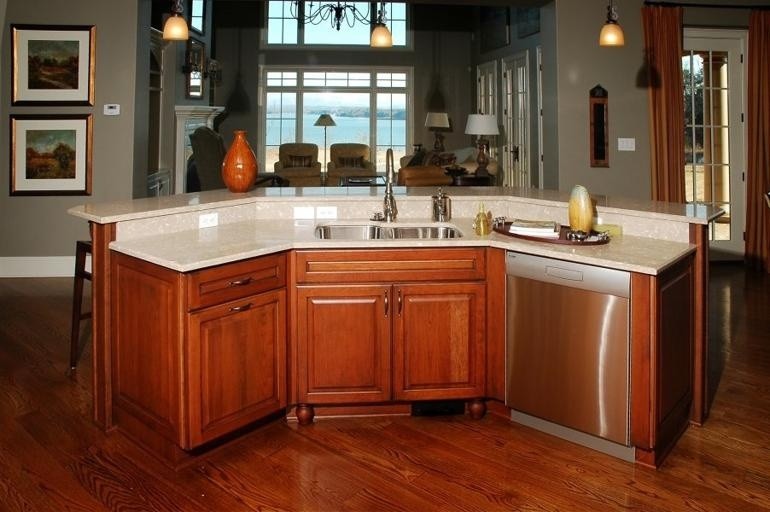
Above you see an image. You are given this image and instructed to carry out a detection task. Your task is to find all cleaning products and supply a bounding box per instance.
[431,188,450,222]
[476,200,490,237]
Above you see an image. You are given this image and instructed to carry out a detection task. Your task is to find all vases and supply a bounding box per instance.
[222,129,258,193]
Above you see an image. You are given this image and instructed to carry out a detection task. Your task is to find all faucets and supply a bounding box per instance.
[383,149,396,221]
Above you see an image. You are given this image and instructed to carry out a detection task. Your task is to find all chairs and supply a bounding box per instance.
[327,143,376,186]
[274,143,321,186]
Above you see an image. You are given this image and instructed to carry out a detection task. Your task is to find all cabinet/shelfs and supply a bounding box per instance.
[111,249,291,473]
[291,247,505,426]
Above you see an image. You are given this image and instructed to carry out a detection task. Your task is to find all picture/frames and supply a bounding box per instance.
[479,13,511,53]
[9,113,93,196]
[10,23,96,106]
[186,36,206,99]
[515,6,539,39]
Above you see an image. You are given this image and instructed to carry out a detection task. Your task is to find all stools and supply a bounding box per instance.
[70,240,92,368]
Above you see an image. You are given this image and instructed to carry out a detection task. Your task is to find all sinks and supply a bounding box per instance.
[313,223,464,240]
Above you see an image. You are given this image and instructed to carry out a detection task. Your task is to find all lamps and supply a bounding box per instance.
[599,0,625,47]
[464,114,500,176]
[424,112,449,165]
[290,0,393,48]
[313,114,336,176]
[163,0,189,41]
